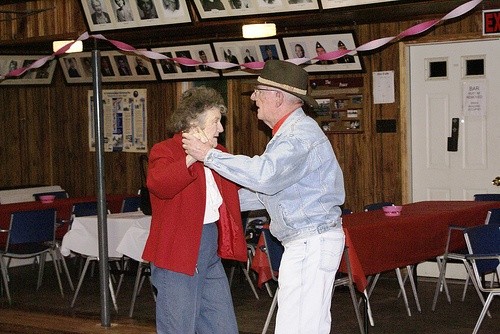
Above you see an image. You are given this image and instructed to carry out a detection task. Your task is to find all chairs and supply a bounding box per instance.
[0,191,157,318]
[240,194,500,334]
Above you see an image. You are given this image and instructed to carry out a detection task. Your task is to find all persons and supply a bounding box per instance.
[142,86,248,334]
[89,0,184,25]
[64,45,277,78]
[6,59,53,80]
[182,59,346,334]
[295,41,356,66]
[200,0,312,13]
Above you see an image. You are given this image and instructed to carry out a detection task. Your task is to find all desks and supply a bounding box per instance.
[60,210,159,312]
[251,201,500,334]
[0,185,69,204]
[0,194,123,269]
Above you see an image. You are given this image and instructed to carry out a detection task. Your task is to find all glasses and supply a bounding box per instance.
[253,88,280,94]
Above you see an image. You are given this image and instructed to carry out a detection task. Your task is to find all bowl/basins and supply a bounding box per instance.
[383,206,402,216]
[39,195,56,203]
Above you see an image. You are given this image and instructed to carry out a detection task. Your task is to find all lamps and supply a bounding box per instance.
[53,39,83,53]
[242,22,276,38]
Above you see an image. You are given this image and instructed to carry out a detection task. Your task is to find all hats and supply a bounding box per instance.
[199,51,205,55]
[265,46,272,51]
[316,41,323,48]
[240,59,319,108]
[337,41,345,47]
[246,49,249,52]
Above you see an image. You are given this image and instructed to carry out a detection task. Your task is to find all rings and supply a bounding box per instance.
[184,144,186,149]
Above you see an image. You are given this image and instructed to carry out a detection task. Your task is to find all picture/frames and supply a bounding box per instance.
[483,9,500,36]
[0,0,423,89]
